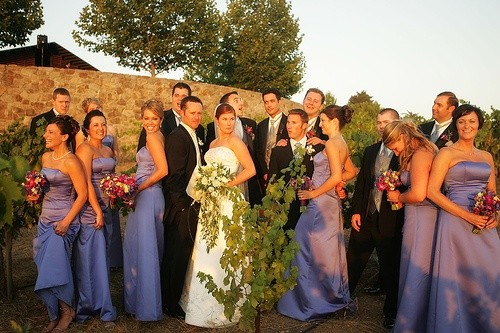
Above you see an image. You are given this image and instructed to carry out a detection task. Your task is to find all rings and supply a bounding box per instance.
[301,195,302,198]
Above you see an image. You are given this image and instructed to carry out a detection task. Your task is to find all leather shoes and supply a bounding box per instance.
[364,284,389,294]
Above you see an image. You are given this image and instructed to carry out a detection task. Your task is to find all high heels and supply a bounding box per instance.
[41,307,76,333]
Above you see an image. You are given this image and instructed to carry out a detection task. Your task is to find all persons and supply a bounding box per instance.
[201,89,360,322]
[130,81,256,333]
[29,87,125,333]
[347,90,500,333]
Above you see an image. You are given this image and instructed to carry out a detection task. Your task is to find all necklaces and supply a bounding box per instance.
[460,145,475,158]
[51,149,71,161]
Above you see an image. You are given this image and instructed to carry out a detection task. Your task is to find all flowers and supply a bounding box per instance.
[472,191,500,234]
[306,145,315,161]
[441,130,453,142]
[307,126,317,138]
[100,173,138,216]
[244,123,255,140]
[20,169,48,206]
[193,162,243,254]
[374,169,404,210]
[290,176,315,212]
[197,137,204,146]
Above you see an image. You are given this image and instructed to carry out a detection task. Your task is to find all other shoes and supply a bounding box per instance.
[383,314,396,328]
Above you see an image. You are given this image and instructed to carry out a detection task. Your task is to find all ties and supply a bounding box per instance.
[174,113,181,123]
[430,120,452,143]
[269,114,282,139]
[378,144,389,165]
[293,143,302,161]
